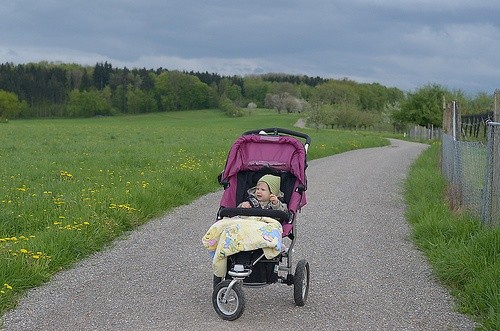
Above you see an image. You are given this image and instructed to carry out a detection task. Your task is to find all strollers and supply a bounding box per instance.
[212,127,310,321]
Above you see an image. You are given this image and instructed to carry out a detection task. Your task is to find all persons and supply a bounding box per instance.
[237,174,288,224]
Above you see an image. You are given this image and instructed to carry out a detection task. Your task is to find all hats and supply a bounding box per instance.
[258,174,281,195]
